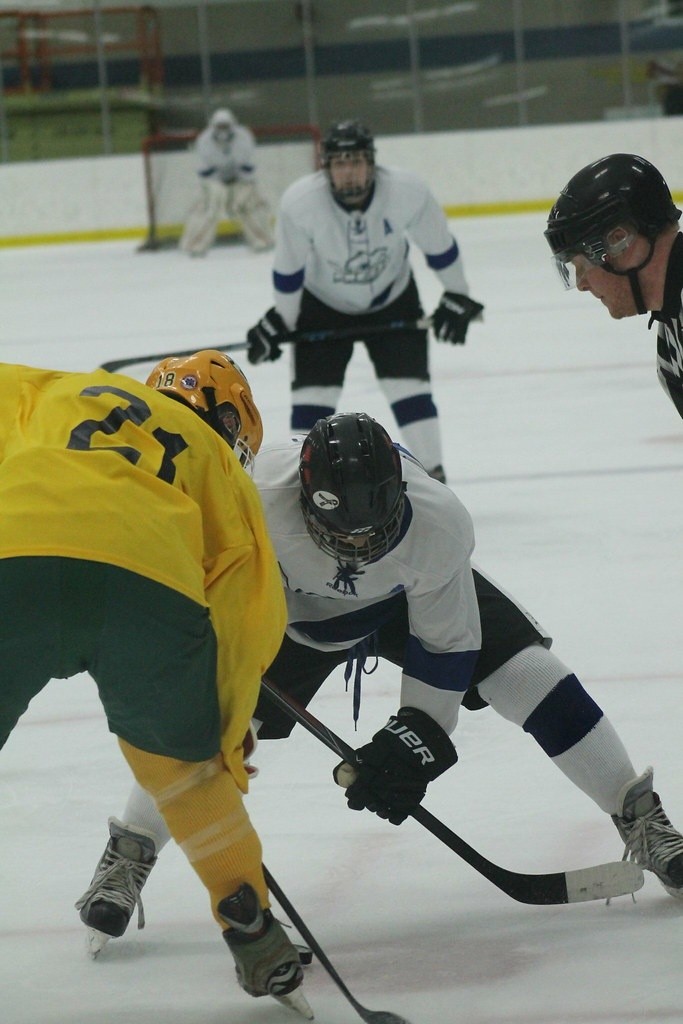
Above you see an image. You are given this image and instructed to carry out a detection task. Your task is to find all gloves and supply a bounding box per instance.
[429,291,484,345]
[333,706,458,826]
[246,307,289,365]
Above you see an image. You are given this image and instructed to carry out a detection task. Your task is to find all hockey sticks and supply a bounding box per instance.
[260,673,647,907]
[98,309,485,374]
[262,862,408,1024]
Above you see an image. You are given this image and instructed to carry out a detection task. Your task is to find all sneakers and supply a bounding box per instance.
[605,765,683,905]
[219,882,314,1021]
[74,815,160,961]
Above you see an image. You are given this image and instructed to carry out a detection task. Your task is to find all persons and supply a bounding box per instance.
[180,108,274,260]
[657,48,683,115]
[544,154,683,418]
[74,413,683,964]
[0,349,315,1022]
[247,121,471,484]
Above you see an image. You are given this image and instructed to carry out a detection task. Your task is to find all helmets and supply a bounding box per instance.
[213,109,235,143]
[145,350,264,481]
[298,412,408,571]
[319,118,376,204]
[544,153,682,292]
